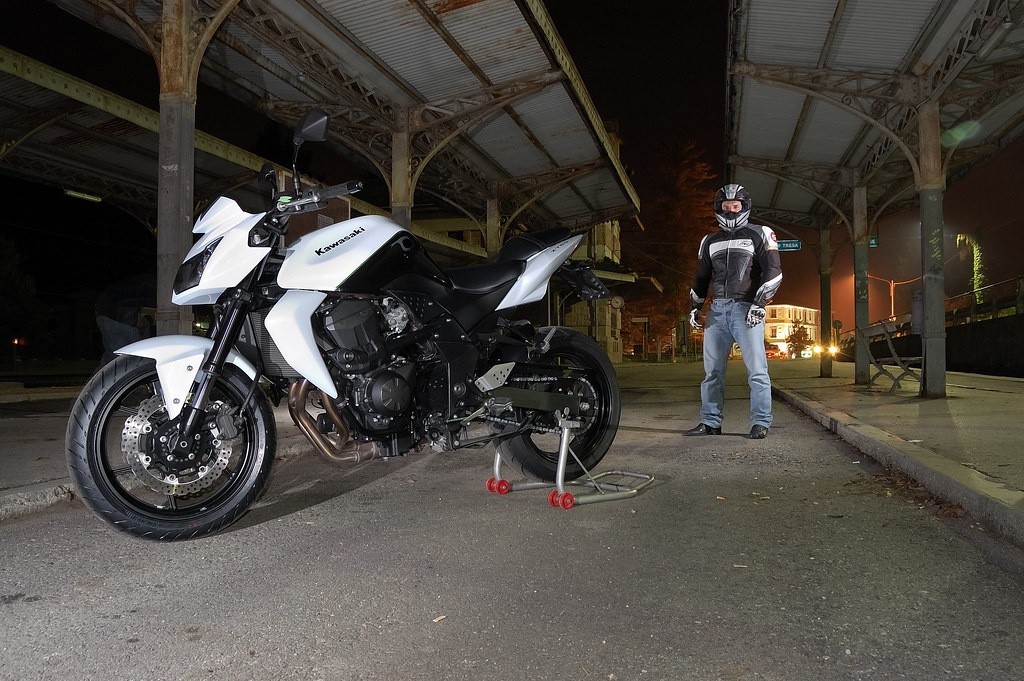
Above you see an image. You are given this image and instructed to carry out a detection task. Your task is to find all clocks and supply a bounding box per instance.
[611,295,624,309]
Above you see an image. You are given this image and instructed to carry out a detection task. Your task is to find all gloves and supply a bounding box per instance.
[745,302,766,328]
[689,309,704,331]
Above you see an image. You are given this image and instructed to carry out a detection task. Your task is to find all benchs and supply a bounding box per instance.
[855,321,923,392]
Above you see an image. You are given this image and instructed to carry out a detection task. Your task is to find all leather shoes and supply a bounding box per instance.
[682,423,721,436]
[749,425,768,438]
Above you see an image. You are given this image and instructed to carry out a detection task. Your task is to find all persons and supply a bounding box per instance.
[686,184,782,438]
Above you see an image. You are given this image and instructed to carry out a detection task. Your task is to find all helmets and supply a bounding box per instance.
[713,183,752,233]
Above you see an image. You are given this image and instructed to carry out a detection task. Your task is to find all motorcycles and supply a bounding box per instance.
[65,105,654,543]
